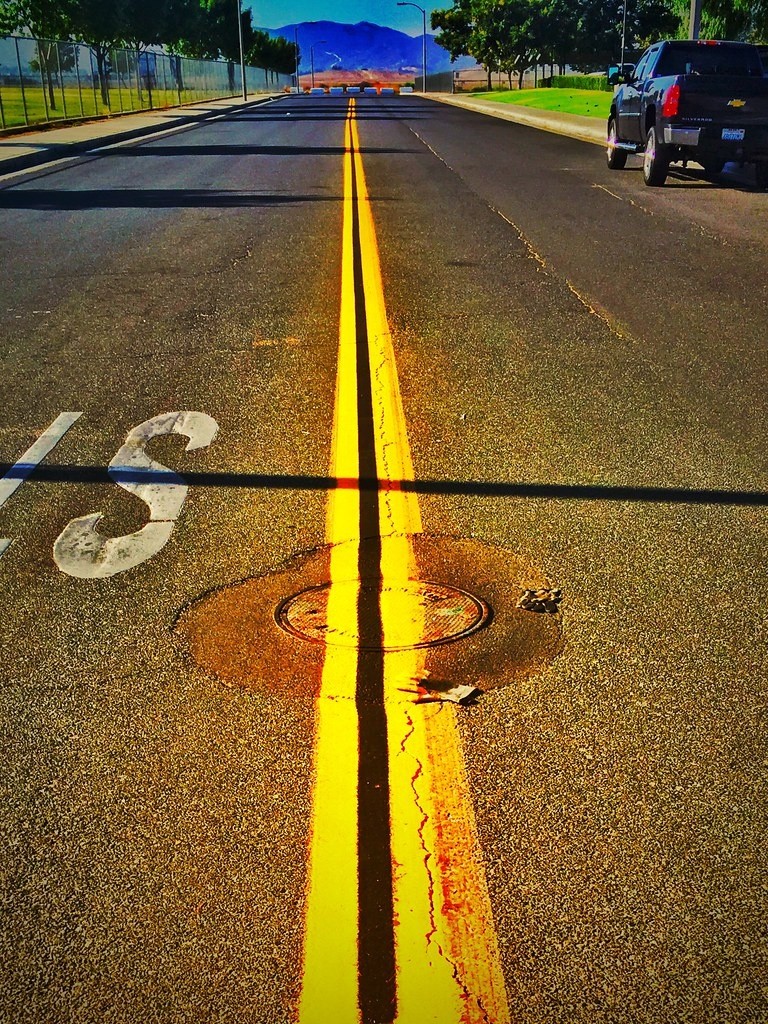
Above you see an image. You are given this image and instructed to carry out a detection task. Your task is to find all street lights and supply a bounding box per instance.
[397,2,426,94]
[295,21,318,94]
[309,40,327,88]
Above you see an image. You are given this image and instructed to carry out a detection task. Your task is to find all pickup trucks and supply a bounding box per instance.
[607,39,768,186]
